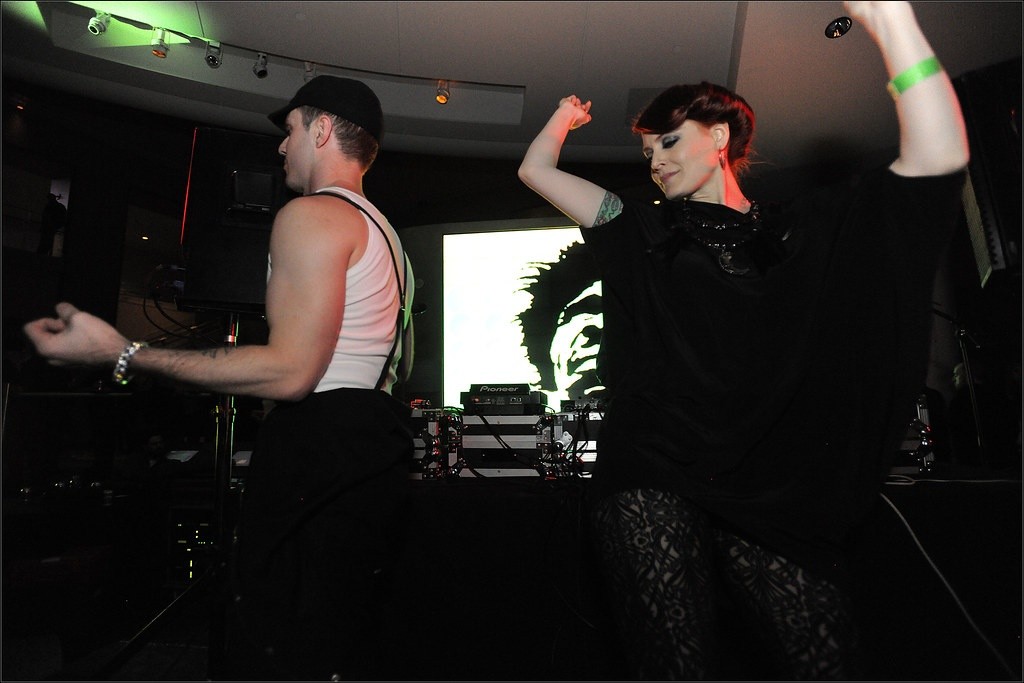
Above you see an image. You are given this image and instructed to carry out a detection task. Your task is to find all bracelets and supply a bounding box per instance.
[888,55,942,102]
[113,341,149,385]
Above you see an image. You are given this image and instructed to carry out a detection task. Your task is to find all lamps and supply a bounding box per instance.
[252,60,269,80]
[151,29,170,58]
[88,12,111,36]
[435,80,450,104]
[205,41,224,69]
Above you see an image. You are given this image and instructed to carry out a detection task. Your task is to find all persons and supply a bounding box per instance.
[25,76,416,682]
[517,3,969,682]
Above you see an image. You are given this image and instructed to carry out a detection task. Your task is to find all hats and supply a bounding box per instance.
[267,75,385,145]
